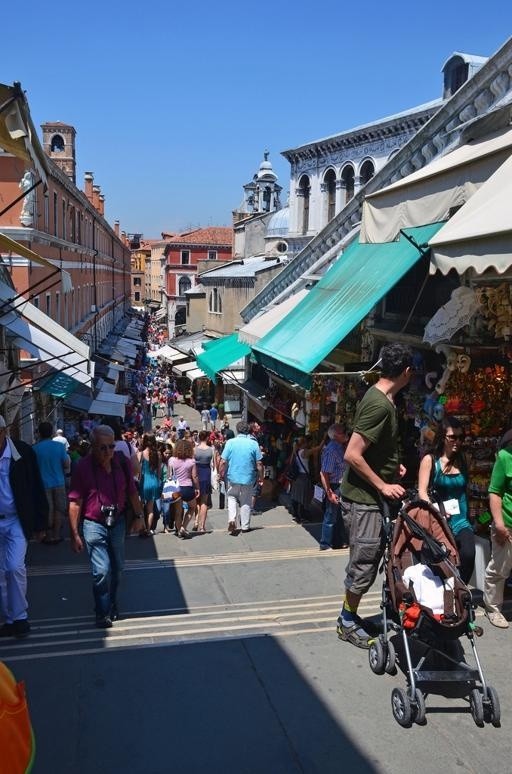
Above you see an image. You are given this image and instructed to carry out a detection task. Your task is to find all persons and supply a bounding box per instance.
[127,320,218,439]
[335,340,416,650]
[31,421,91,544]
[0,415,50,640]
[417,415,477,587]
[68,425,144,627]
[482,444,512,627]
[99,417,273,538]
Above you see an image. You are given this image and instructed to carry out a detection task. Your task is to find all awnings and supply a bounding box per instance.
[42,313,146,423]
[0,233,96,392]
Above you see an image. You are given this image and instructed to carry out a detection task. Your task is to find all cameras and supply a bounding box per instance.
[101,504,120,529]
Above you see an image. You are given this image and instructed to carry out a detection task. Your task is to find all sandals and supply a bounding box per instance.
[337,619,378,648]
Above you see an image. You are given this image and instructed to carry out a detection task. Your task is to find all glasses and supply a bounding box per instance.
[449,435,465,441]
[101,445,115,450]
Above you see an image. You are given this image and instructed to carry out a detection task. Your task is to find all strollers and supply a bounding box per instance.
[366,493,506,735]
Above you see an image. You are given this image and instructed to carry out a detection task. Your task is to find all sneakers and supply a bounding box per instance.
[97,612,118,627]
[0,619,30,637]
[485,610,509,628]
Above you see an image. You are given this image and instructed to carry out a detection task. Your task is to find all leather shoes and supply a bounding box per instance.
[228,522,251,533]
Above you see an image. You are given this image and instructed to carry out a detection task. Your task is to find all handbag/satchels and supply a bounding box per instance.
[161,457,181,503]
[126,503,143,537]
[285,465,298,480]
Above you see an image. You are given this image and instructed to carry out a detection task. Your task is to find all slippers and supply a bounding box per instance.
[144,527,205,537]
